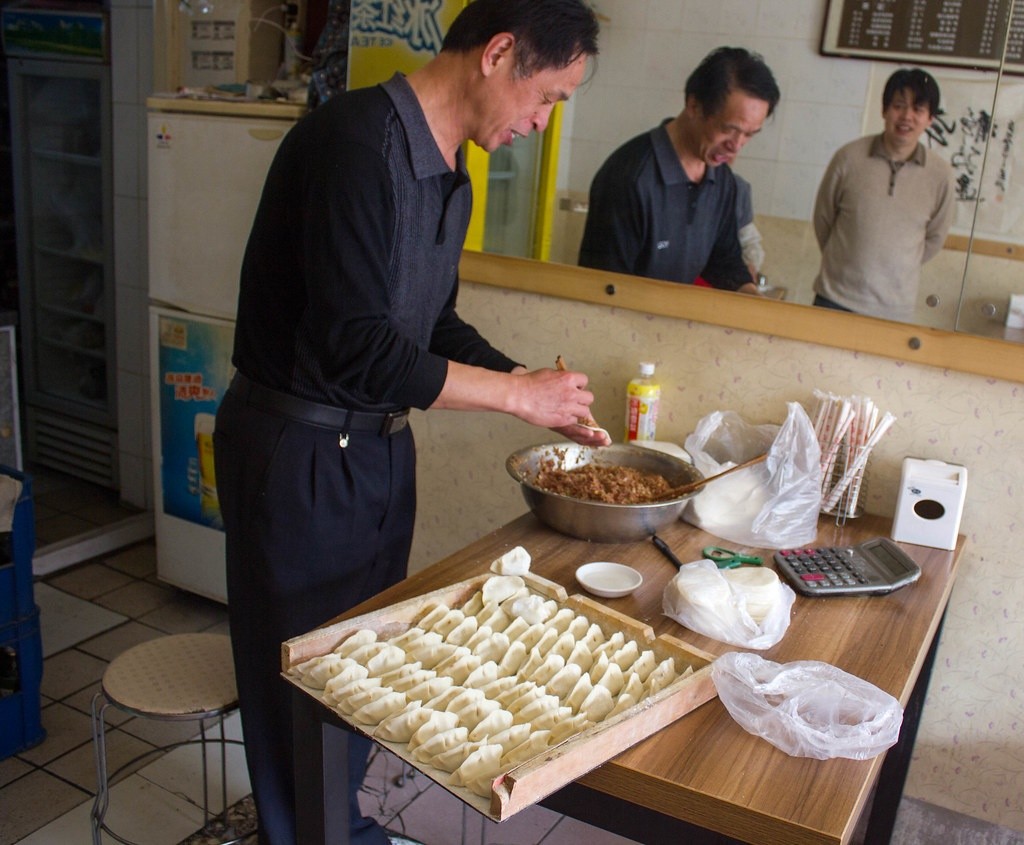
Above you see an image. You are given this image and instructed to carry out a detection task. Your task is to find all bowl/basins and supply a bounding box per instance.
[506,441,705,542]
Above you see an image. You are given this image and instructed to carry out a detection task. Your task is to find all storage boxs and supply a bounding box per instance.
[0,606,49,762]
[0,464,39,631]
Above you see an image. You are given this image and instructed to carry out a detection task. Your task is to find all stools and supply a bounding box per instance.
[89,631,257,845]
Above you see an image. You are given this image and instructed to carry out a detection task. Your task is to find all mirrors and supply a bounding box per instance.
[457,0,1024,384]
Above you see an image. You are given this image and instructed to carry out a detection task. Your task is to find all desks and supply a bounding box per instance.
[285,508,966,845]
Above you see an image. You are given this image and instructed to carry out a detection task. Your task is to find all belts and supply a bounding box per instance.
[227,374,412,438]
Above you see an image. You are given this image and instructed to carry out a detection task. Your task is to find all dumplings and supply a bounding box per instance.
[286,545,695,799]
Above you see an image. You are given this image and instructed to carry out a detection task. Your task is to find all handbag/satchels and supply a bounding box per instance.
[660,558,797,651]
[678,400,822,550]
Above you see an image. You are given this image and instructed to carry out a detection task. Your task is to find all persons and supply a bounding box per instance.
[580,44,779,292]
[813,69,956,325]
[219,0,619,845]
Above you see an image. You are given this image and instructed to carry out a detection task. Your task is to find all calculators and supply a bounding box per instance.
[772,535,923,598]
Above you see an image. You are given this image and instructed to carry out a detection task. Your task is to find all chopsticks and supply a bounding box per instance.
[555,355,567,371]
[811,392,896,515]
[655,452,767,501]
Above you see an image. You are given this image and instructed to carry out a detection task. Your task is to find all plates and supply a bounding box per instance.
[575,562,643,598]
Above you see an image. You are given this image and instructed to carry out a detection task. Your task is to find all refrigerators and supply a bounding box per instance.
[0,7,118,491]
[342,0,567,263]
[145,97,304,604]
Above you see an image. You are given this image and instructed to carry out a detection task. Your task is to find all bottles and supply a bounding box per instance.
[623,361,659,444]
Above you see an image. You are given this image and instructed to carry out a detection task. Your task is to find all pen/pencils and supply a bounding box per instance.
[653,534,683,571]
